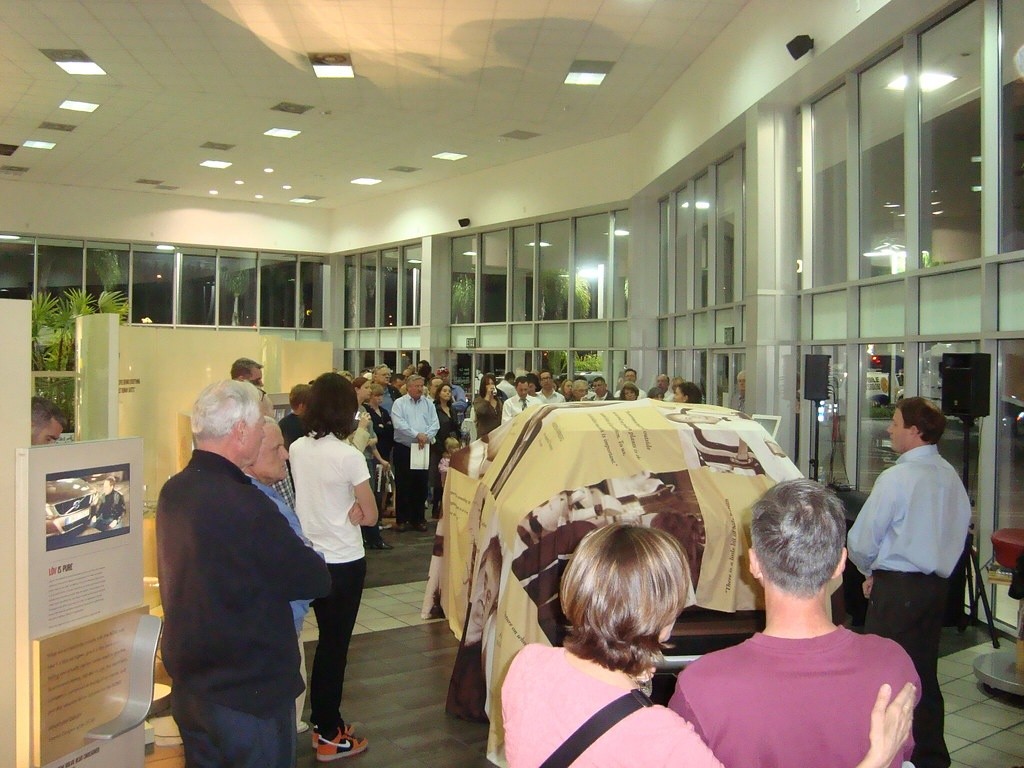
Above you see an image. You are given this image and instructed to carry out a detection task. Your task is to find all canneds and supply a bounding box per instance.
[492,385,497,395]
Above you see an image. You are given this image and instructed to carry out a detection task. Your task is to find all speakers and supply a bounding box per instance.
[803,353,832,403]
[938,352,991,418]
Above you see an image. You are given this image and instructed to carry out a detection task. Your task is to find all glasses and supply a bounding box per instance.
[258,388,267,402]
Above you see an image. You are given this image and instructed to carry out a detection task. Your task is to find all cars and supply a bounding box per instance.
[46,478,100,539]
[440,402,856,717]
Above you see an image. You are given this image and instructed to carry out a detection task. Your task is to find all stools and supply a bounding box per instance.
[973,528,1024,697]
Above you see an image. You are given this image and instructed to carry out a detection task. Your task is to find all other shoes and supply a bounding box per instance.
[432,512,440,518]
[395,523,406,532]
[415,524,427,531]
[370,540,392,549]
[297,721,309,733]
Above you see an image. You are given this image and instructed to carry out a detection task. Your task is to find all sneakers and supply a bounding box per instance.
[317,727,369,761]
[312,724,354,748]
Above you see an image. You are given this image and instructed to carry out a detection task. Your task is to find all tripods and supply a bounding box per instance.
[944,417,1001,650]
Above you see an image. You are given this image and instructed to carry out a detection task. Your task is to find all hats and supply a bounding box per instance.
[437,366,449,375]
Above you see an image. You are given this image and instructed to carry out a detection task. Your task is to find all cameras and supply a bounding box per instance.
[355,412,363,420]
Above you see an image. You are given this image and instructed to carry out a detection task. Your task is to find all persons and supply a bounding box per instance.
[244,414,323,733]
[155,379,332,768]
[289,373,377,761]
[849,396,972,768]
[279,361,701,551]
[501,521,916,768]
[96,477,125,532]
[669,480,922,768]
[731,370,746,412]
[231,357,265,390]
[255,395,295,505]
[32,397,65,447]
